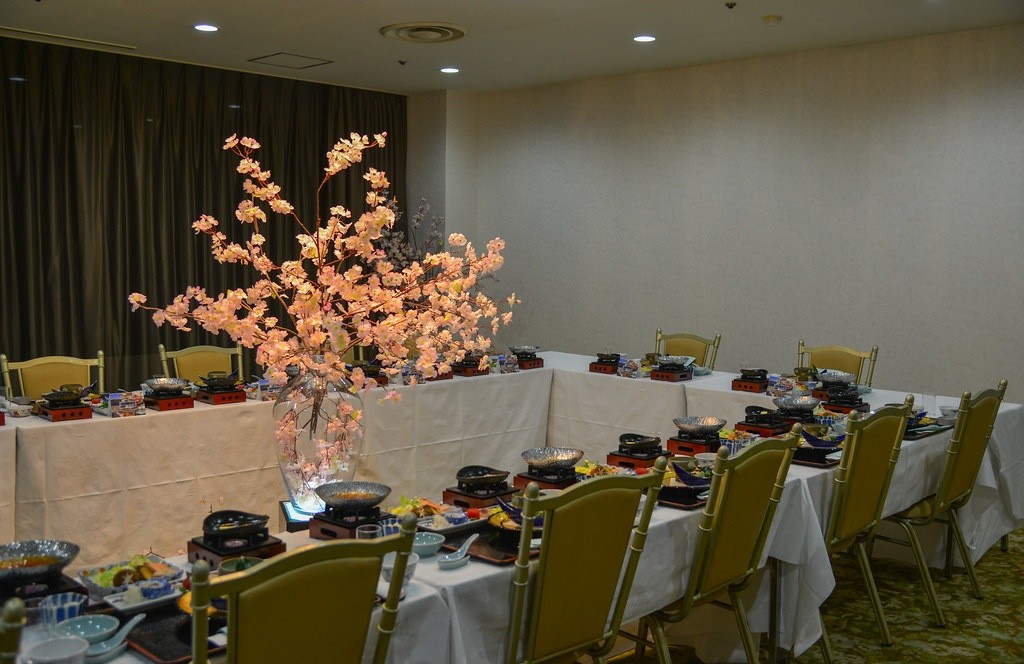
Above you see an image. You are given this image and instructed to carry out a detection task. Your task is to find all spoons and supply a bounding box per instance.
[439,533,477,561]
[85,614,147,656]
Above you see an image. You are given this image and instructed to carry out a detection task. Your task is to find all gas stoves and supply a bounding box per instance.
[0,355,870,617]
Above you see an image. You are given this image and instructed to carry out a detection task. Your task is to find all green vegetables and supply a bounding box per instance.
[696,466,713,476]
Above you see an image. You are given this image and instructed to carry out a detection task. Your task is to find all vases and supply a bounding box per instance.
[273,354,368,516]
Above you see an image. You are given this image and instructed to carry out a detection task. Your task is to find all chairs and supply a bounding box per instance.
[634,421,803,664]
[159,340,244,385]
[503,454,668,664]
[818,393,915,664]
[652,328,721,371]
[0,349,105,402]
[866,377,1008,627]
[189,514,419,664]
[795,340,878,389]
[314,334,365,366]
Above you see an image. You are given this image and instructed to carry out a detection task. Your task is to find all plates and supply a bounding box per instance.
[662,477,710,490]
[488,510,545,532]
[104,585,183,615]
[177,590,229,623]
[418,512,486,534]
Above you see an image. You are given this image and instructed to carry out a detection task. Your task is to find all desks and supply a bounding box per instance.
[0,349,1024,664]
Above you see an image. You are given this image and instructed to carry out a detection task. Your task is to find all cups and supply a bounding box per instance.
[354,525,381,540]
[39,592,88,632]
[377,519,402,536]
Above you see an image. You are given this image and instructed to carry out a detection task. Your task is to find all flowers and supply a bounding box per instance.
[126,131,522,507]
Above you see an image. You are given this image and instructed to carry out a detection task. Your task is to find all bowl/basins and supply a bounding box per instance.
[217,556,263,578]
[940,405,958,417]
[55,615,118,641]
[381,551,419,585]
[696,451,718,469]
[29,636,88,664]
[908,411,928,425]
[665,456,696,474]
[512,491,546,507]
[410,532,445,557]
